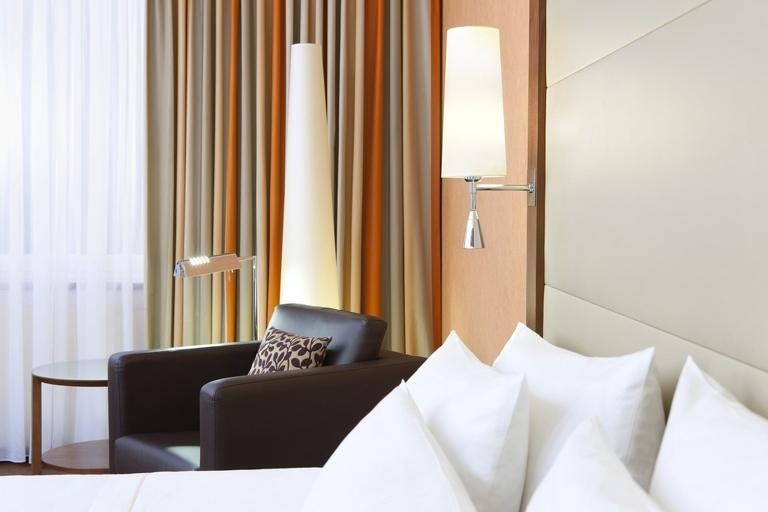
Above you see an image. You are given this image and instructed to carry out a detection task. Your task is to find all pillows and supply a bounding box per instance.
[492,319,665,512]
[320,379,476,512]
[404,330,526,512]
[648,357,768,512]
[247,327,331,375]
[526,415,660,512]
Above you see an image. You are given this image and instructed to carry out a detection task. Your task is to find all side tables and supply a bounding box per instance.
[31,359,109,474]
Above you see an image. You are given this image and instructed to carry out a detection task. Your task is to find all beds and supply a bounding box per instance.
[0,467,363,511]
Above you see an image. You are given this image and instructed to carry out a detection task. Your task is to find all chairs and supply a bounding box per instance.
[109,303,427,470]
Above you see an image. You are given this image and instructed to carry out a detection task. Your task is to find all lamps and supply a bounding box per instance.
[440,25,537,248]
[172,252,258,342]
[278,44,343,314]
